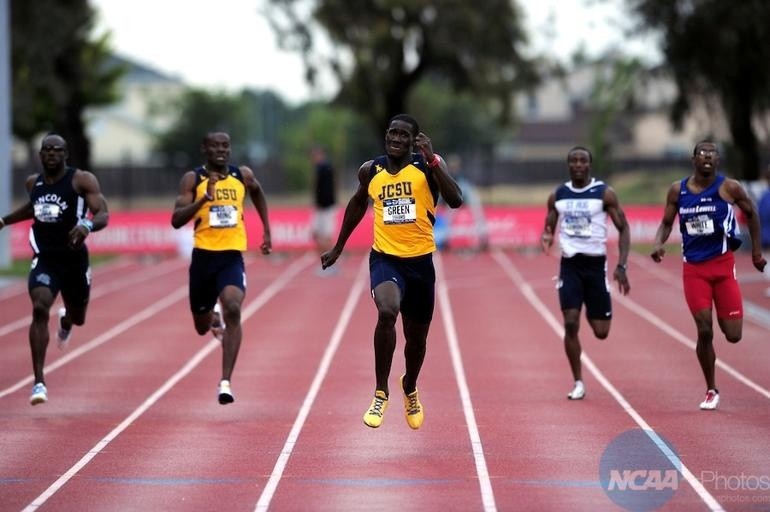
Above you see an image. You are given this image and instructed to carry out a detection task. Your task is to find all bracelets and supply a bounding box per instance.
[617,262,628,272]
[204,192,214,201]
[78,218,96,232]
[0,217,6,227]
[753,256,764,263]
[426,153,441,169]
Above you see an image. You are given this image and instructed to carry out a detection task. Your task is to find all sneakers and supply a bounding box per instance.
[29,382,49,406]
[700,387,720,412]
[216,377,236,405]
[363,390,389,429]
[567,382,586,400]
[400,372,424,429]
[53,308,72,352]
[211,302,227,341]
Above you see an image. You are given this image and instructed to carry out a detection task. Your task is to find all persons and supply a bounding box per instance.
[649,139,769,411]
[307,144,339,278]
[538,145,633,401]
[170,129,275,407]
[320,112,465,431]
[0,130,112,407]
